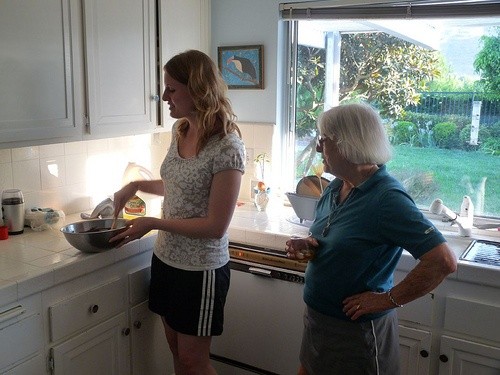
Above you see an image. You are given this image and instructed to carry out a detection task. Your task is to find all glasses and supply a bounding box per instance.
[315,135,328,148]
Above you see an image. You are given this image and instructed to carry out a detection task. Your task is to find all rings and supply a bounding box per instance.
[352,304,360,312]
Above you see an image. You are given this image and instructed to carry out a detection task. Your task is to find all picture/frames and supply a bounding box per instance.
[217,44,264,90]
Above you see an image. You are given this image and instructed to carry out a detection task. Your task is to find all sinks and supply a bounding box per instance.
[459,239,500,267]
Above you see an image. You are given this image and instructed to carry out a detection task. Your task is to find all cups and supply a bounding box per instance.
[0,220,8,240]
[291,232,313,263]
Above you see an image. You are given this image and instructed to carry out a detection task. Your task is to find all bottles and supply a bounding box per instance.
[255,182,269,211]
[123,162,156,219]
[2,189,24,235]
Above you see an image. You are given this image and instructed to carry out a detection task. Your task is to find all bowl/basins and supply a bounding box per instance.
[60,218,127,253]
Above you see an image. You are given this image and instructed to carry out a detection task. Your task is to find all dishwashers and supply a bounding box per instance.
[210,259,306,375]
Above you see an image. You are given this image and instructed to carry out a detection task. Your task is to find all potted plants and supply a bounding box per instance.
[253,152,272,214]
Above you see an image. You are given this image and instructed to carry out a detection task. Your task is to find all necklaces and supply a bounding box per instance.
[322,166,376,237]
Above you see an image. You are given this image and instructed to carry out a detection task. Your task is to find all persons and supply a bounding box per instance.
[285,104,457,375]
[108,51,245,375]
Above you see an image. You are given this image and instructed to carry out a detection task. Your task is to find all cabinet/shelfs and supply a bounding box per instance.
[0,250,175,375]
[394,270,500,375]
[0,0,211,149]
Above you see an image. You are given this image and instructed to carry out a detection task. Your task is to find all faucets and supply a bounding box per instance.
[456,174,487,216]
[429,195,474,237]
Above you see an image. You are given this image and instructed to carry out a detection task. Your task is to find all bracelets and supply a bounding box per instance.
[387,288,403,308]
[132,181,138,191]
